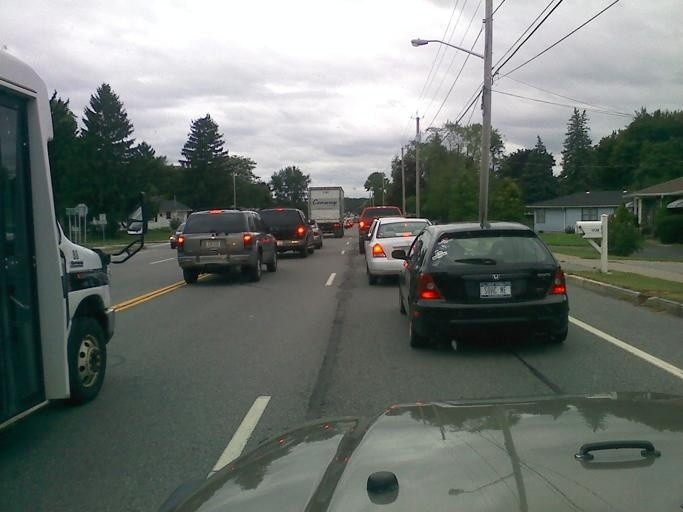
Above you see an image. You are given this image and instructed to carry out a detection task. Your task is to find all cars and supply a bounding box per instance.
[393,218,569,350]
[310,223,323,249]
[362,217,435,285]
[158,390,683,512]
[169,222,186,249]
[343,216,360,229]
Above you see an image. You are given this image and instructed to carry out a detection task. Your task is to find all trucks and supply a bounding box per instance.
[0,49,148,433]
[307,186,345,238]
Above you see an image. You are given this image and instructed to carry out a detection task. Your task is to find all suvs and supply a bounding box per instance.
[260,208,316,257]
[357,206,405,255]
[176,210,278,284]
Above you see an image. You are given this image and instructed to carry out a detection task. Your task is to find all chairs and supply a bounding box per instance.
[443,241,514,263]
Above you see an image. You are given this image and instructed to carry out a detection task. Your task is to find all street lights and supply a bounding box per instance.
[411,38,484,60]
